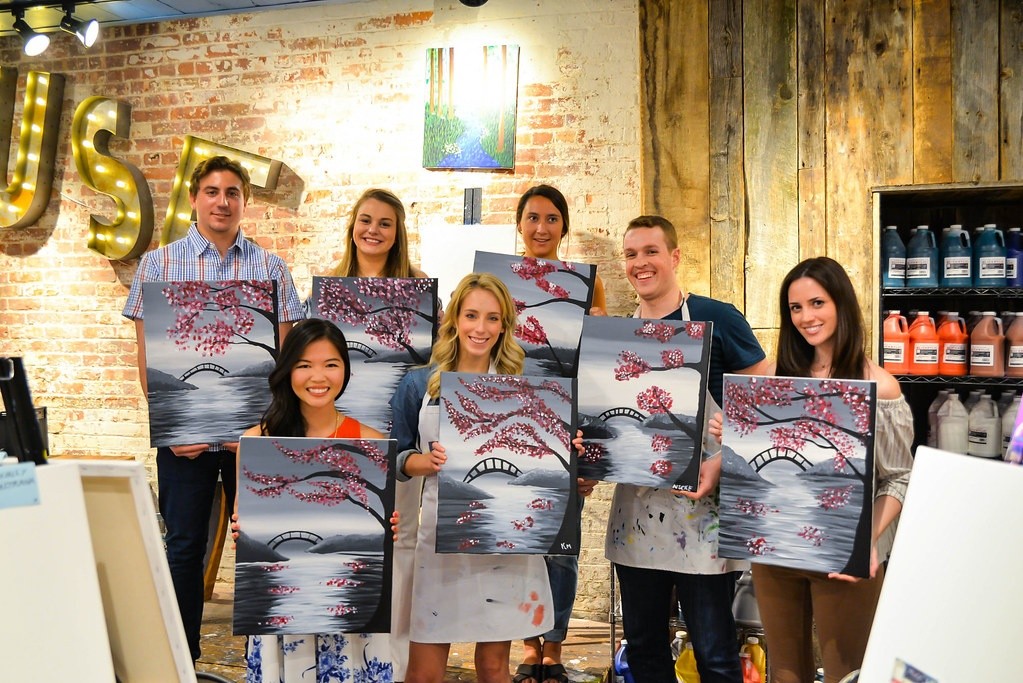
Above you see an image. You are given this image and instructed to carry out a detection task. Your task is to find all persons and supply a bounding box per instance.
[121,156,303,669]
[512,185,604,683]
[230,318,393,683]
[604,216,767,683]
[388,274,587,683]
[709,257,915,683]
[303,188,443,319]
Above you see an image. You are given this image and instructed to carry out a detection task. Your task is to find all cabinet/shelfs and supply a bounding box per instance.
[870,183,1023,465]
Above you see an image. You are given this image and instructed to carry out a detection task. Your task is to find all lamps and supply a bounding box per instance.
[12,8,51,56]
[59,0,99,49]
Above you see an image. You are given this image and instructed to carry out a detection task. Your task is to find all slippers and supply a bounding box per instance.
[514,662,543,683]
[541,663,569,683]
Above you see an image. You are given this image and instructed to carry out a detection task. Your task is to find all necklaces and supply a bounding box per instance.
[822,365,825,368]
[333,408,339,437]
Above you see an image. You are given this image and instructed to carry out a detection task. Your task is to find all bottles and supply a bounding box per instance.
[814,668,824,683]
[615,639,635,683]
[881,223,1023,287]
[738,652,760,683]
[740,637,766,683]
[670,630,688,661]
[674,642,700,683]
[883,309,1023,377]
[928,388,1021,457]
